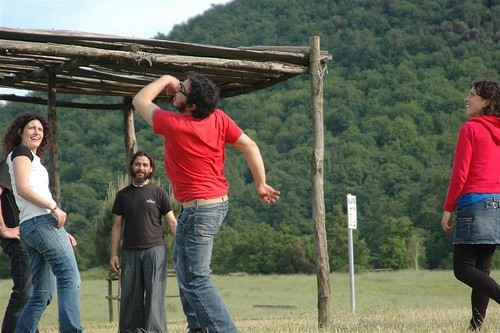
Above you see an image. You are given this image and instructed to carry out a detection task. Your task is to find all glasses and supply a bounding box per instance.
[179,84,187,97]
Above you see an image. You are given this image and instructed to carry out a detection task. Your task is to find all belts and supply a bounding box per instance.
[181,195,228,208]
